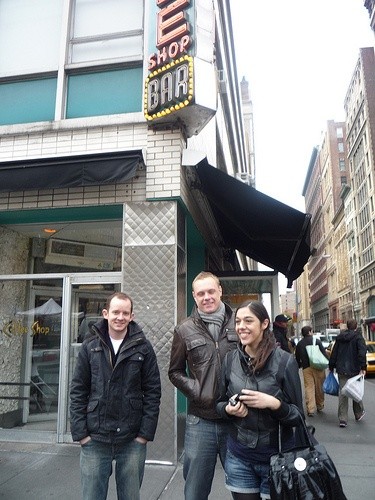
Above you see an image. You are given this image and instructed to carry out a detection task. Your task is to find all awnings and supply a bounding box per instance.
[209,164,310,289]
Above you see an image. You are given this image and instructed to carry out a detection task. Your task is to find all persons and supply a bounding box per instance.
[69,291,162,500]
[83,321,96,341]
[271,314,295,354]
[329,319,367,426]
[296,326,331,416]
[168,272,241,500]
[216,300,305,500]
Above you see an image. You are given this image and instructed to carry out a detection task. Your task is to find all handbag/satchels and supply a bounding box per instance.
[268,403,347,500]
[305,334,330,370]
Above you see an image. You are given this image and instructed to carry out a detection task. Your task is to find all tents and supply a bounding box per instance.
[17,299,83,350]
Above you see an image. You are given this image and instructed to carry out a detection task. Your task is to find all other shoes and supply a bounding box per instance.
[317,407,323,412]
[339,421,347,427]
[308,413,314,416]
[355,410,366,422]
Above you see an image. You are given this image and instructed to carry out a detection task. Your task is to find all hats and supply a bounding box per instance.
[275,314,292,322]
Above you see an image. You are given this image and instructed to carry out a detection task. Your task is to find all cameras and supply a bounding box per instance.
[229,394,240,406]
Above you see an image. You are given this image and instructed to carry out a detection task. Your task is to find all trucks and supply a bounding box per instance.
[325,328,341,341]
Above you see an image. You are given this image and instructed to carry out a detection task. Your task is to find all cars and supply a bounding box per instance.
[365,340,375,380]
[326,340,335,360]
[290,331,329,348]
